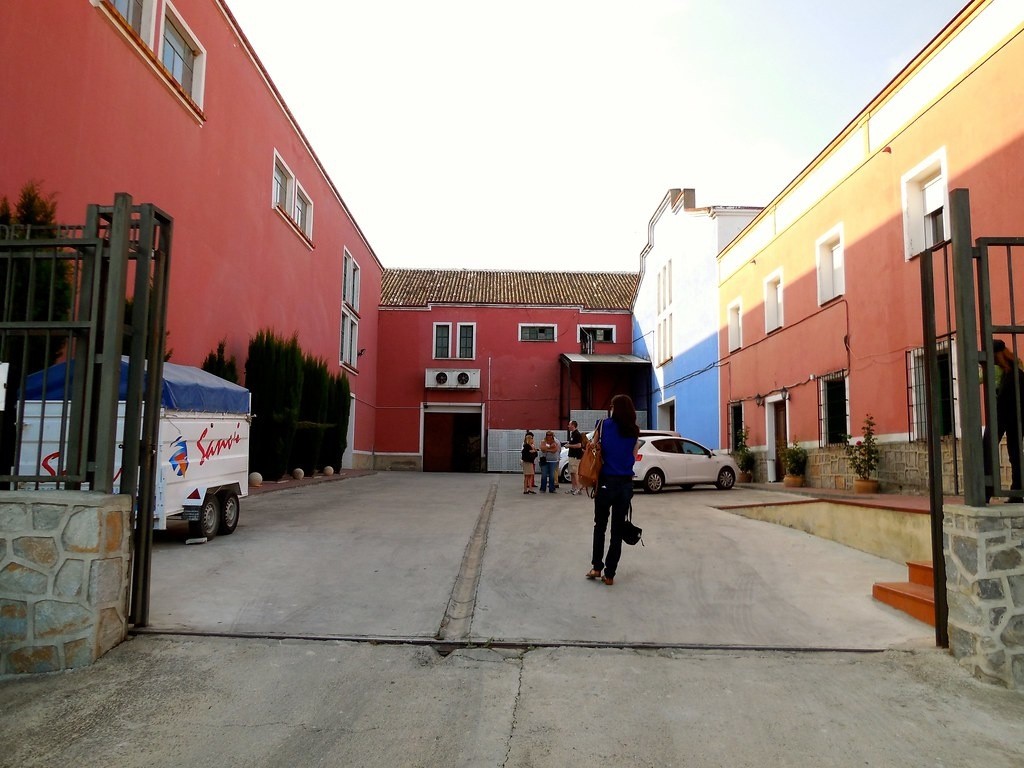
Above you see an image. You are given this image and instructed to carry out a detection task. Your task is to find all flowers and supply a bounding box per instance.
[840,410,880,480]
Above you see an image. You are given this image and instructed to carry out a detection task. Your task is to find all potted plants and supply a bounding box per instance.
[729,424,755,484]
[775,432,808,487]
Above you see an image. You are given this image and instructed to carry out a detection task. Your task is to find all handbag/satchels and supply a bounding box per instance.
[539,456,546,467]
[621,501,645,546]
[578,419,604,499]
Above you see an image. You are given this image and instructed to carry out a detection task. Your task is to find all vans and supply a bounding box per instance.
[557,429,681,483]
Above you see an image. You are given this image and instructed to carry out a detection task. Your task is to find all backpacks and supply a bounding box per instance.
[579,431,589,450]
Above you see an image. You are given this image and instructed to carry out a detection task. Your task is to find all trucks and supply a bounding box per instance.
[11,351,253,540]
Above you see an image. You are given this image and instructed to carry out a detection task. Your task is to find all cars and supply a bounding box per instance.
[632,435,742,492]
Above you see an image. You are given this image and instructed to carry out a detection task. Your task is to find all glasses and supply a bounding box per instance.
[531,438,533,439]
[546,434,552,436]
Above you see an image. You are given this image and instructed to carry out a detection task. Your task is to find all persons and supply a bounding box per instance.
[522,435,538,494]
[521,430,538,487]
[564,421,583,494]
[983,339,1024,503]
[551,431,561,488]
[539,431,558,493]
[586,395,640,585]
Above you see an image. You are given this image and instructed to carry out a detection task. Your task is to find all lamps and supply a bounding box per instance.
[754,393,765,407]
[357,348,365,355]
[779,385,790,401]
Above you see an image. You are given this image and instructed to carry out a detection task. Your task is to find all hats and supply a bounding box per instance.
[526,431,534,436]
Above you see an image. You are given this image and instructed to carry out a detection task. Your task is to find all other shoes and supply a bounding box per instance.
[553,489,558,493]
[524,490,537,494]
[547,484,559,488]
[532,484,538,487]
[564,488,582,495]
[585,568,601,577]
[600,574,613,585]
[538,489,546,493]
[526,485,529,488]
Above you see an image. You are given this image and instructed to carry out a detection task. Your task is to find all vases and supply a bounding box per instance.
[854,477,880,494]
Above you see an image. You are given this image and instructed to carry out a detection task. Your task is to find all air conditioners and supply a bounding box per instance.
[450,369,480,389]
[424,368,450,388]
